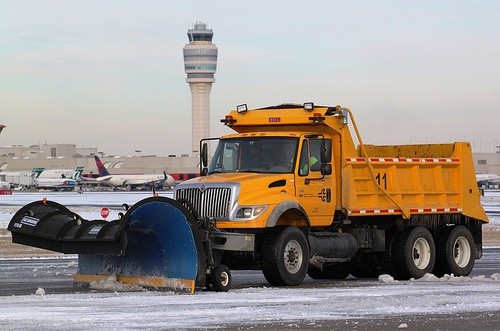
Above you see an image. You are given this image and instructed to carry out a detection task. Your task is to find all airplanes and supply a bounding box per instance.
[31,154,176,191]
[475,173,500,188]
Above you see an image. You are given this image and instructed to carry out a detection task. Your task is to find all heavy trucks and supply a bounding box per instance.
[174,101,489,286]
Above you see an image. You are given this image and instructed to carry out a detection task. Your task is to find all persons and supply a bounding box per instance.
[286,139,321,174]
[241,143,271,170]
